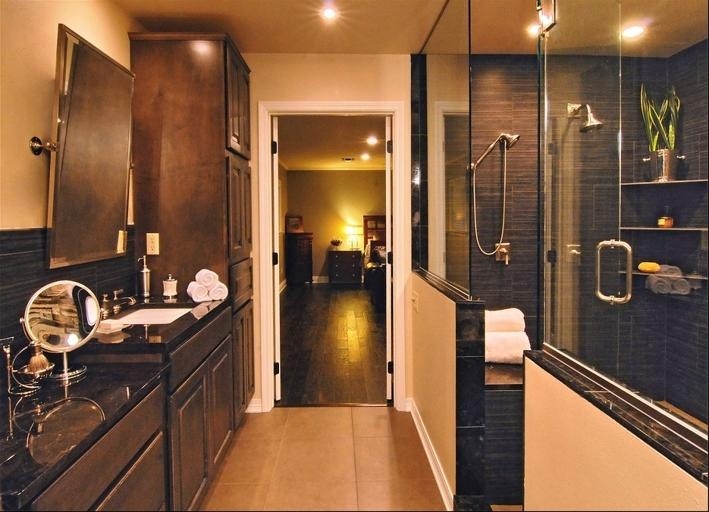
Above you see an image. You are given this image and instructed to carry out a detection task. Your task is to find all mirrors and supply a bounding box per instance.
[25,386,105,472]
[46,22,135,270]
[19,278,102,386]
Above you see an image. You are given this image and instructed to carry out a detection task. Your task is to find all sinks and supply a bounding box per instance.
[109,302,201,324]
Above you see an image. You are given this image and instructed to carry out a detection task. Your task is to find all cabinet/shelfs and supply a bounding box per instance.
[223,41,252,161]
[166,305,238,511]
[232,257,255,413]
[284,233,313,284]
[0,361,167,509]
[226,154,255,263]
[620,177,707,408]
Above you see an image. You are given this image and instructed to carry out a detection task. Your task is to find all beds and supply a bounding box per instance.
[362,215,386,311]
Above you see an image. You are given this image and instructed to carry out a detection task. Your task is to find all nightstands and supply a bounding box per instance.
[327,250,362,288]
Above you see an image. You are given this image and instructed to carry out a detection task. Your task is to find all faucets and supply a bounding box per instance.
[98,293,137,315]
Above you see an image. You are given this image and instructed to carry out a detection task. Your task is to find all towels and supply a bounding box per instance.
[186,268,228,302]
[644,263,692,296]
[484,308,532,364]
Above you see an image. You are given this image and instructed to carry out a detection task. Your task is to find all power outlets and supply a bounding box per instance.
[146,232,160,256]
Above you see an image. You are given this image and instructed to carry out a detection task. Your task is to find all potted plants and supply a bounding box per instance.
[640,81,681,182]
